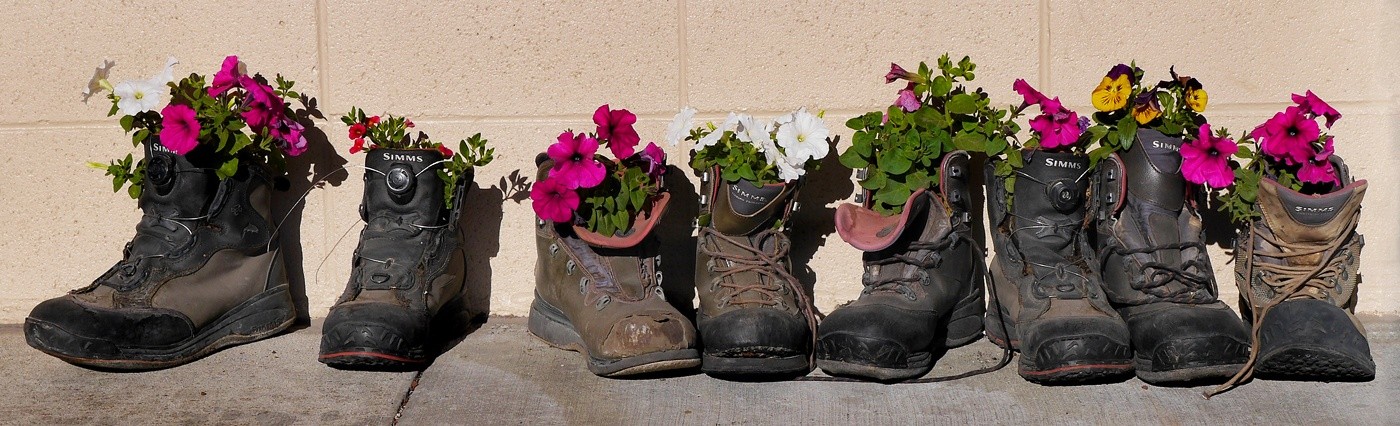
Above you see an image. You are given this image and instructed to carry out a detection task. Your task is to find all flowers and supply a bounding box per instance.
[68,42,1367,239]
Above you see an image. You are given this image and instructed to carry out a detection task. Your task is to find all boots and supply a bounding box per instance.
[692,162,816,377]
[528,151,700,377]
[984,149,1134,381]
[317,146,474,366]
[792,150,1011,384]
[24,149,295,369]
[1199,154,1377,401]
[1095,124,1251,383]
